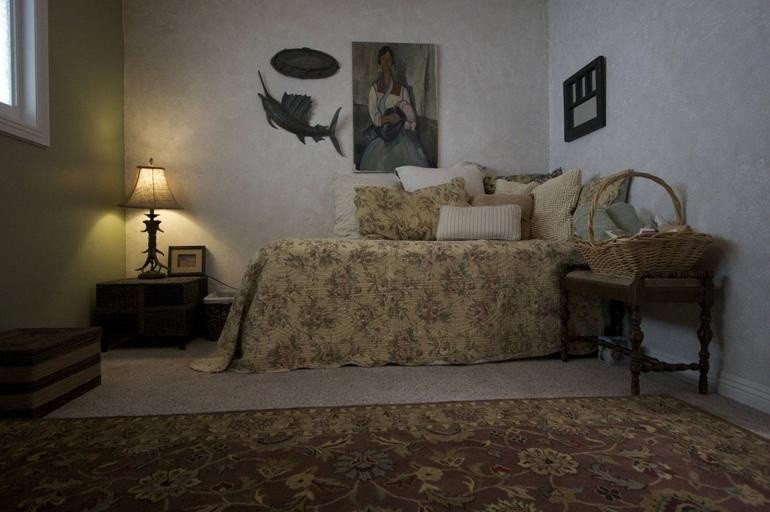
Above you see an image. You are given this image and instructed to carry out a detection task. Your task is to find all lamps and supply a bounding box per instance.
[113,156,185,279]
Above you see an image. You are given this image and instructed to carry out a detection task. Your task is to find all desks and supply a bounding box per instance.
[557,266,717,398]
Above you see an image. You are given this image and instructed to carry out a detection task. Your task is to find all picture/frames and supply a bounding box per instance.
[167,245,206,276]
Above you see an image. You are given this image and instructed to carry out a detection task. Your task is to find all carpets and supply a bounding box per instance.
[0,390,770,511]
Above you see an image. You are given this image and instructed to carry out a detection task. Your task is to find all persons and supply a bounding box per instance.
[359,46,429,170]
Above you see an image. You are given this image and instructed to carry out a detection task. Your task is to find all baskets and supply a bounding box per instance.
[576,171,713,280]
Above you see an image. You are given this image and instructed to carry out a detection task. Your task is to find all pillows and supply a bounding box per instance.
[529,168,582,242]
[483,167,562,195]
[471,192,536,242]
[354,177,470,241]
[494,178,541,195]
[394,161,486,203]
[576,167,634,212]
[436,204,522,240]
[334,172,404,240]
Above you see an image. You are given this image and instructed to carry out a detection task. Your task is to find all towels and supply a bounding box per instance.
[604,200,645,237]
[571,200,622,244]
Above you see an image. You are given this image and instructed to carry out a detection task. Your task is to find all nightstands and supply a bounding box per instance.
[97,275,209,352]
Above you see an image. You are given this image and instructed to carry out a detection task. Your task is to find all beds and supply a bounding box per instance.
[188,168,636,373]
